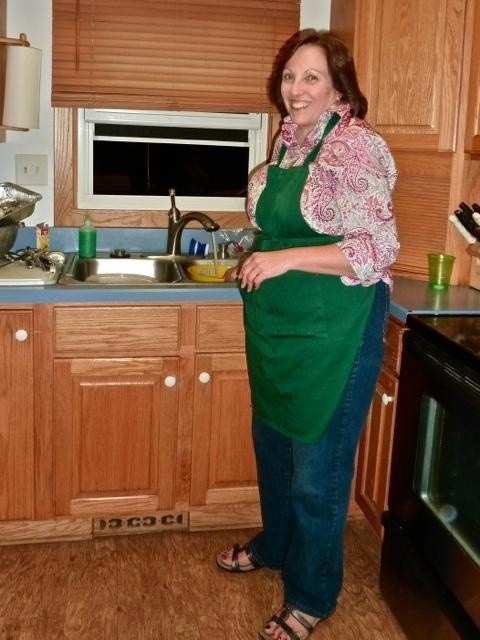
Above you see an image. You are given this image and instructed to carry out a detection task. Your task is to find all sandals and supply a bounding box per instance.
[260,602,336,640]
[216,542,264,573]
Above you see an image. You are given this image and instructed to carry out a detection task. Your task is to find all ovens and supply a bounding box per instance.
[381,330,480,634]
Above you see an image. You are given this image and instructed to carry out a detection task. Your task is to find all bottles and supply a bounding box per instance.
[78,211,97,258]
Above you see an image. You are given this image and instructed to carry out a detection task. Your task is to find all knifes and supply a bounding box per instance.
[448,199,480,246]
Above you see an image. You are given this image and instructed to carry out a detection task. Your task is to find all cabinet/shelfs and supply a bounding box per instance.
[330,0,467,154]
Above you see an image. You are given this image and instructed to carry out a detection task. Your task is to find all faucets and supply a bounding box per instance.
[217,240,241,259]
[164,188,221,254]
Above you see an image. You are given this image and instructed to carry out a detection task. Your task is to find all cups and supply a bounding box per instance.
[427,253,455,292]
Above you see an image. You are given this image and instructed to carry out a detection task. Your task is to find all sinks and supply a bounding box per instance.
[178,250,242,287]
[58,251,184,288]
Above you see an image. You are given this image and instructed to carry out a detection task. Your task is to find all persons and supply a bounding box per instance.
[216,28,400,640]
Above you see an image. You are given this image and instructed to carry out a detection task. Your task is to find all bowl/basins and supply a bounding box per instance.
[187,264,234,282]
